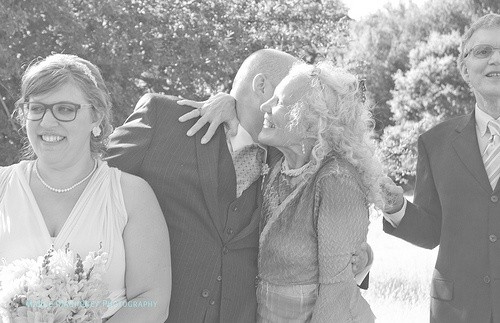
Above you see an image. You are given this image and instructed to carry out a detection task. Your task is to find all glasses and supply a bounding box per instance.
[19,97,92,122]
[463,44,500,59]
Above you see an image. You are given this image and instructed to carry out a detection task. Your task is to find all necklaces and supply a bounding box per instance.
[34,156,97,192]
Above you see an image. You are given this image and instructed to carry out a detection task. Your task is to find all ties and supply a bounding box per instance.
[230,144,264,199]
[481,120,500,192]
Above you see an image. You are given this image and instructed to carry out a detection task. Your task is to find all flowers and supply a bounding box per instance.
[0,239,111,323]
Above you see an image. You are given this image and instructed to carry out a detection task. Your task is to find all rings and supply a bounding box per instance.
[197,106,203,116]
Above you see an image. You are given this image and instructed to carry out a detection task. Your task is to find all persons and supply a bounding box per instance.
[368,14,500,323]
[176,61,378,323]
[0,54,172,323]
[101,48,375,323]
